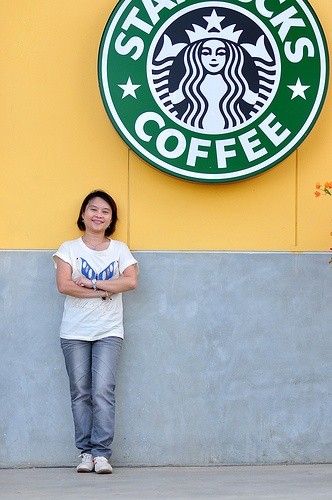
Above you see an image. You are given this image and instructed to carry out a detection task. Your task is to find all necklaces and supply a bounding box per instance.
[82,236,110,250]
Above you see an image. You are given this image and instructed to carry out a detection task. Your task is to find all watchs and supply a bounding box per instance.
[91,278,97,290]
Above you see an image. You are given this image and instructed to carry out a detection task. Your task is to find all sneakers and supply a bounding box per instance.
[77,454,94,472]
[94,456,113,473]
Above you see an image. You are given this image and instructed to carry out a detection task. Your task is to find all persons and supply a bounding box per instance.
[50,188,140,475]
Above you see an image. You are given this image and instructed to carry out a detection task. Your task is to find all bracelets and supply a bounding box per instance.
[99,289,108,300]
